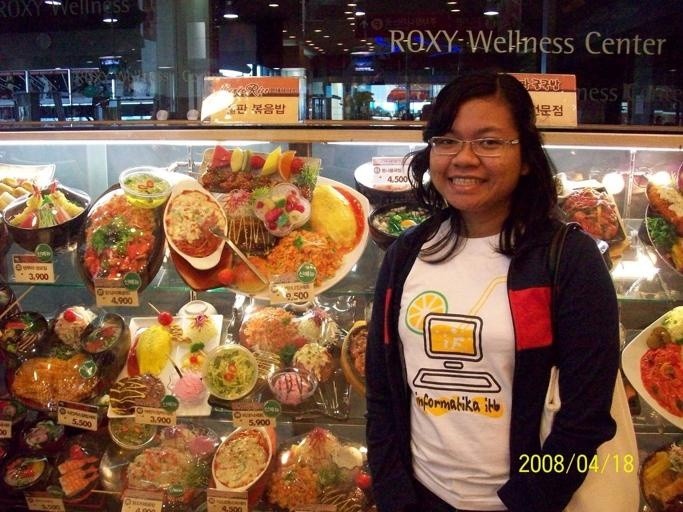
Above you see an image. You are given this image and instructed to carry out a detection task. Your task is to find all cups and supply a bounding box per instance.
[12,89,41,122]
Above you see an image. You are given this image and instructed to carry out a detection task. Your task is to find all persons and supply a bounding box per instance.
[363,69,621,512]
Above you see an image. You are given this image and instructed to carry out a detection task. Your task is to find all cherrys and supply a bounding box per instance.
[291,158,303,173]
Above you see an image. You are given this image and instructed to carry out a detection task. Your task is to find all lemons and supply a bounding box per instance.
[230,147,243,172]
[406,290,448,334]
[261,145,281,176]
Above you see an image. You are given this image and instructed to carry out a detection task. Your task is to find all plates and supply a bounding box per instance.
[620,303,683,432]
[107,313,224,418]
[76,182,166,296]
[163,179,229,272]
[169,176,372,302]
[2,310,132,413]
[211,426,273,492]
[97,420,221,509]
[266,435,374,511]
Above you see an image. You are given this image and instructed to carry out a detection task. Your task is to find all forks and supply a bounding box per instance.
[207,223,269,285]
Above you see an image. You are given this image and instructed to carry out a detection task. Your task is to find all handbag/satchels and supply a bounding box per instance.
[541,360,639,511]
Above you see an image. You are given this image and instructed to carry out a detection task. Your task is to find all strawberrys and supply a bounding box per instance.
[251,155,266,169]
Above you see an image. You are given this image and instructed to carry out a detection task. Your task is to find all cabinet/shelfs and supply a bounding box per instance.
[0,68,683,512]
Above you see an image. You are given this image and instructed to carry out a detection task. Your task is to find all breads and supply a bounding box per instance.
[108,374,164,414]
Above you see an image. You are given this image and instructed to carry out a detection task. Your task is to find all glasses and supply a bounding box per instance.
[430,136,522,157]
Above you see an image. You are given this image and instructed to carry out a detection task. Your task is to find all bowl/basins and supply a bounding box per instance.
[268,366,319,406]
[2,188,93,254]
[19,416,69,455]
[201,343,259,401]
[354,161,431,207]
[119,166,172,209]
[0,449,48,494]
[108,419,159,451]
[367,201,442,252]
[175,299,218,341]
[79,314,125,357]
[0,309,49,360]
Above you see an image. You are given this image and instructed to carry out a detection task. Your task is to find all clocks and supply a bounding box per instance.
[35,32,52,50]
